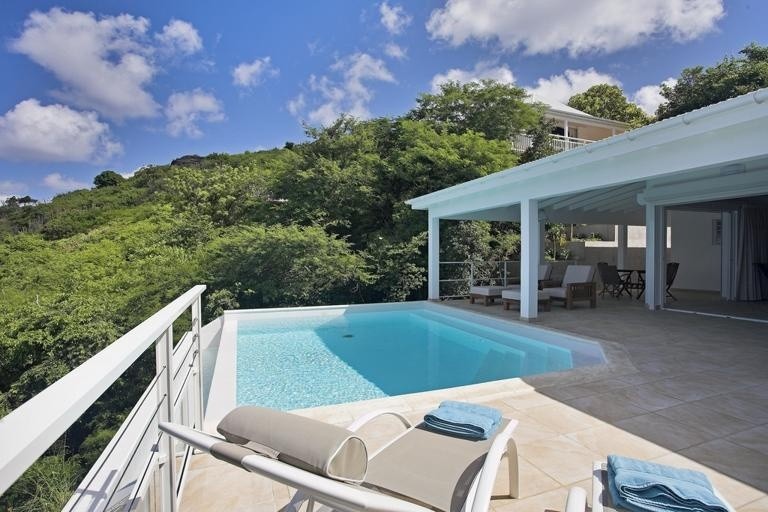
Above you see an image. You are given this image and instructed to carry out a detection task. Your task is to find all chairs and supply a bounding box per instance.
[562,455,736,510]
[598,262,679,302]
[509,264,598,308]
[158,399,521,511]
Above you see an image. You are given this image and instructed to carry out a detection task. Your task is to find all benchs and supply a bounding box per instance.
[469,286,551,313]
